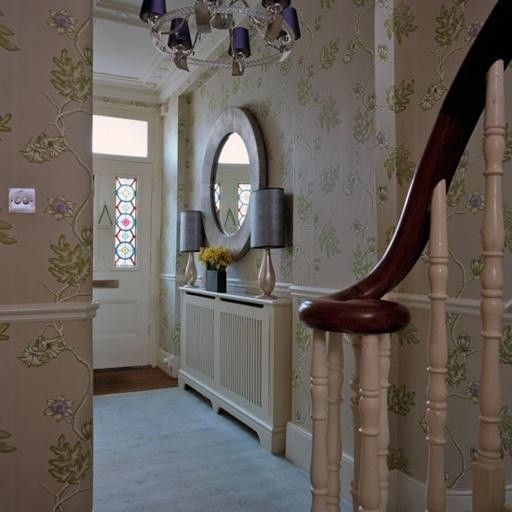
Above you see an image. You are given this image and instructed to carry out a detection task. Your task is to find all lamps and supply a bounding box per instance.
[139,0,301,76]
[178,210,204,288]
[249,188,285,299]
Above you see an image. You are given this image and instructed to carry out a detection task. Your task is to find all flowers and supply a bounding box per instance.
[198,246,232,271]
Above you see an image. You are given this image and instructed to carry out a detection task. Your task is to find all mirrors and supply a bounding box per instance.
[198,104,269,261]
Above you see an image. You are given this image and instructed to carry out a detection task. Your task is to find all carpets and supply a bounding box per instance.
[92,386,355,512]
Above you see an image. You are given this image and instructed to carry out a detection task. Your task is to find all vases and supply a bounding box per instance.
[205,270,227,292]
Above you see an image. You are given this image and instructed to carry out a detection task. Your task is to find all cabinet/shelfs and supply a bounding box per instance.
[176,286,292,455]
